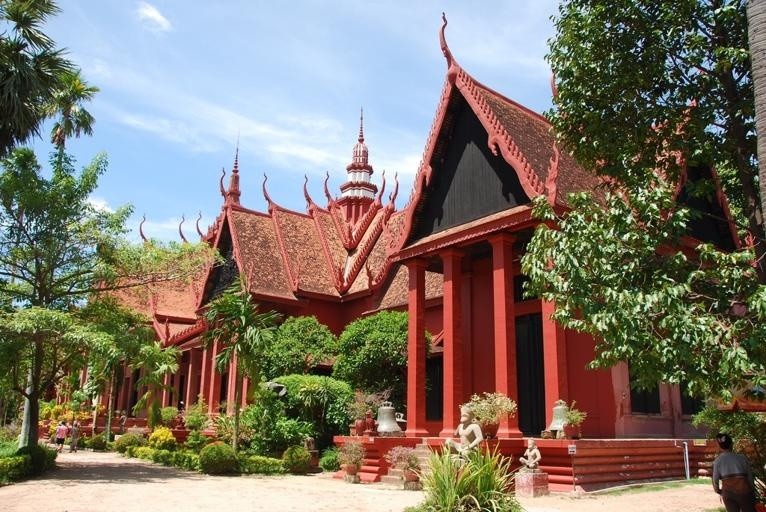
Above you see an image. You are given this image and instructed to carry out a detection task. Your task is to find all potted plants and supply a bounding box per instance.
[458,391,517,439]
[282,445,313,474]
[403,454,422,481]
[562,400,588,440]
[335,440,366,475]
[348,398,378,437]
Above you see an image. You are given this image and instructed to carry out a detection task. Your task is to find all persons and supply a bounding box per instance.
[119,410,127,433]
[712,433,758,512]
[68,420,78,453]
[55,421,68,453]
